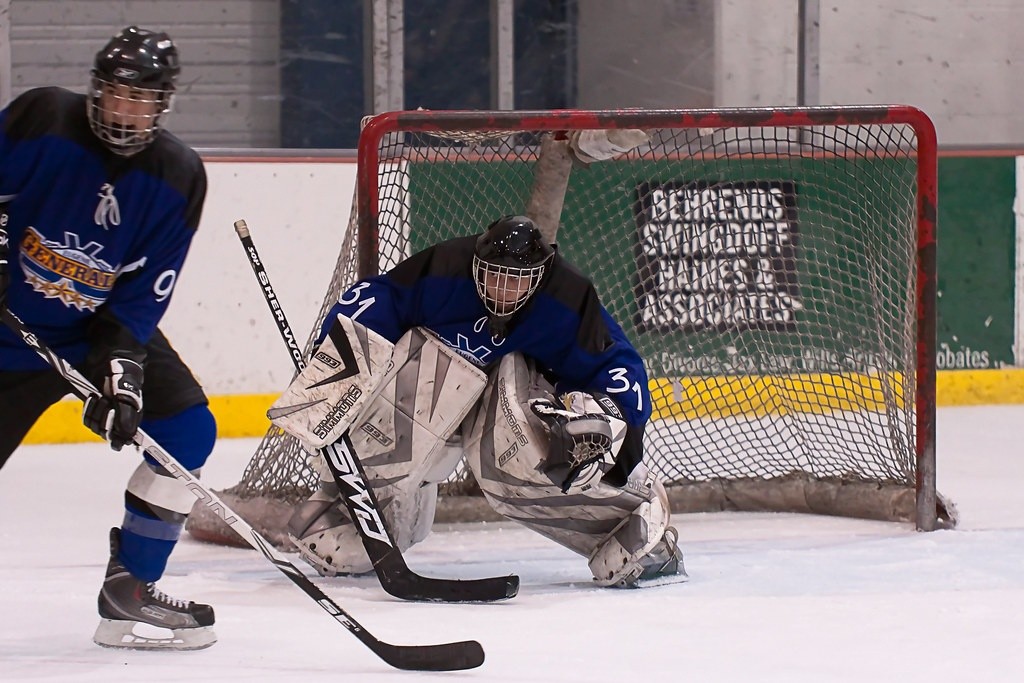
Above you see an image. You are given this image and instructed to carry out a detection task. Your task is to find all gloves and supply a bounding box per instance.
[83,347,147,451]
[0,211,11,305]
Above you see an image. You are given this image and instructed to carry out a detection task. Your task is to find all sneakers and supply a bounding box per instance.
[93,527,218,651]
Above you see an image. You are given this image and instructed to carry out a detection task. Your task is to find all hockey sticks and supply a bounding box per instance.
[230,217,523,608]
[0,302,486,674]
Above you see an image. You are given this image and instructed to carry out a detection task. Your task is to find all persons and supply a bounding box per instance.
[0,25,216,653]
[266,216,686,587]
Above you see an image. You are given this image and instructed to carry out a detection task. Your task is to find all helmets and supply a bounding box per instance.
[86,26,181,156]
[472,214,555,317]
